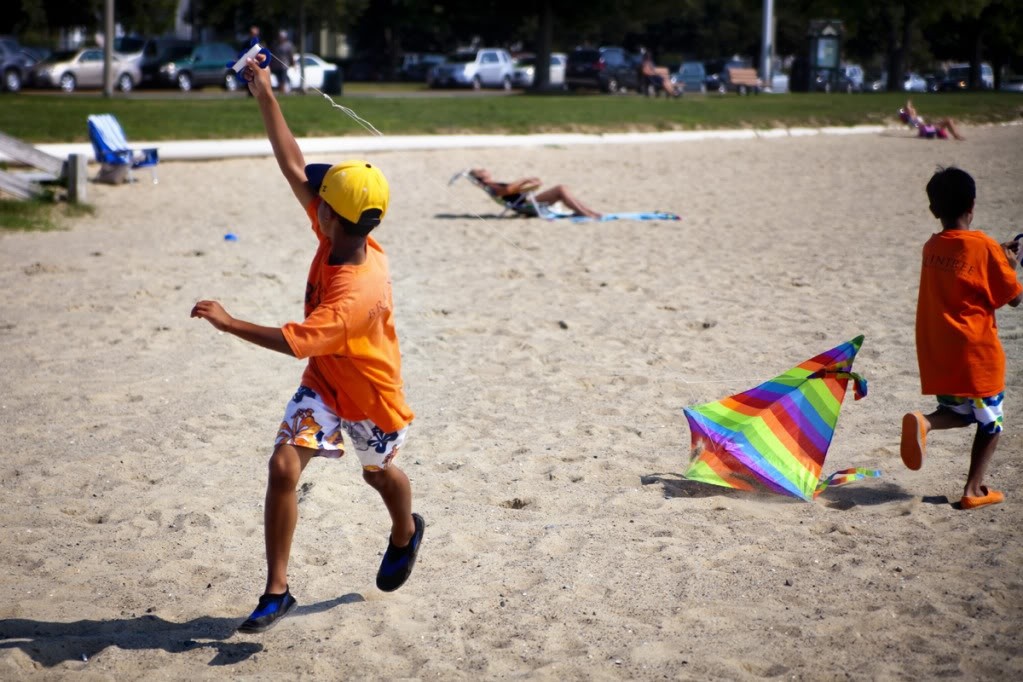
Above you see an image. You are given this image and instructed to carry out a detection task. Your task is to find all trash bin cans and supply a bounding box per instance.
[324,71,341,96]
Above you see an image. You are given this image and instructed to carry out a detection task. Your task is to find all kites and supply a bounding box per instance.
[681,334,882,502]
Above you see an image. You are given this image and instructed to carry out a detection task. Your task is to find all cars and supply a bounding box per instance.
[28,47,142,93]
[816,69,854,94]
[511,52,570,91]
[0,43,33,93]
[426,48,514,91]
[704,62,727,88]
[758,70,788,94]
[1000,74,1023,93]
[867,72,888,87]
[863,77,880,90]
[270,52,337,92]
[395,53,444,71]
[898,74,927,92]
[0,38,40,65]
[112,37,146,60]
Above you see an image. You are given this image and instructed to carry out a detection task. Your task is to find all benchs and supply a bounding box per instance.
[87,112,159,184]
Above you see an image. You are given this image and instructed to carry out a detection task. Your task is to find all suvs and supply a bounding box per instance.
[666,63,706,94]
[140,39,238,91]
[564,46,643,94]
[929,68,983,93]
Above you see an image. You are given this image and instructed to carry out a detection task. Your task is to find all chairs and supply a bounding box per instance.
[449,169,554,218]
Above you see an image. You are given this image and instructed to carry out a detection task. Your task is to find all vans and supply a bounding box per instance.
[838,64,864,92]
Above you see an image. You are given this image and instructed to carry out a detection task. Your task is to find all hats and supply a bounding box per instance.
[304,159,389,224]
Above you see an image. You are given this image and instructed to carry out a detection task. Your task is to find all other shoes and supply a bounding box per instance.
[238,585,298,632]
[900,410,926,470]
[961,486,1004,509]
[377,512,424,592]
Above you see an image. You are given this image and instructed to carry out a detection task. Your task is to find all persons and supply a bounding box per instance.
[900,100,965,141]
[470,169,602,219]
[190,54,426,634]
[642,52,681,96]
[247,26,296,94]
[901,167,1023,509]
